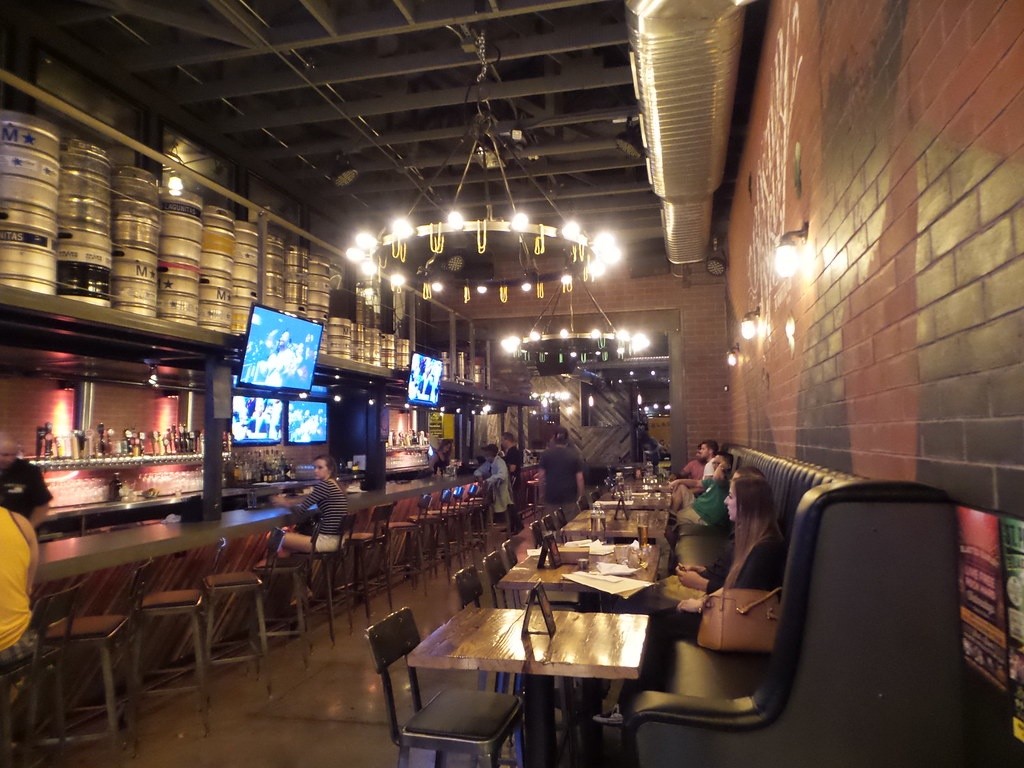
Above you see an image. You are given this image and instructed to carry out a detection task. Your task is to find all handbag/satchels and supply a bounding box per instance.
[696,589,782,653]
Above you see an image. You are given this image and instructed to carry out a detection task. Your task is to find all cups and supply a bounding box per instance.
[636,513,649,551]
[45,477,109,508]
[446,465,456,477]
[136,471,204,496]
[247,490,257,509]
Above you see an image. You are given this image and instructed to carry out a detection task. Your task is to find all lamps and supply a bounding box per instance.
[775,221,808,278]
[705,241,728,277]
[727,343,739,365]
[515,279,635,364]
[333,153,359,188]
[614,123,644,160]
[740,306,761,340]
[343,29,599,303]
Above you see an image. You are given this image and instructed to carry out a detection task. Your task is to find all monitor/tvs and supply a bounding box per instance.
[405,351,443,408]
[230,302,330,446]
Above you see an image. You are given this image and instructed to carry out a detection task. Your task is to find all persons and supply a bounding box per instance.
[0,432,52,755]
[238,327,319,387]
[269,455,349,607]
[406,353,443,403]
[468,444,514,546]
[425,439,453,477]
[288,403,326,442]
[496,433,525,536]
[538,427,585,530]
[592,438,781,724]
[231,398,282,441]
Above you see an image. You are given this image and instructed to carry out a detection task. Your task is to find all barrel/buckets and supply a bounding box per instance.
[0,109,412,373]
[440,351,483,386]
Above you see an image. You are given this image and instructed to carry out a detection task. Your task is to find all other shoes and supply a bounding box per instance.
[512,526,525,534]
[500,524,514,531]
[291,591,312,604]
[591,705,624,725]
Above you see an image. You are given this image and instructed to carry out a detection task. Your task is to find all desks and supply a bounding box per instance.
[497,544,661,767]
[406,605,652,768]
[592,476,672,510]
[559,510,668,545]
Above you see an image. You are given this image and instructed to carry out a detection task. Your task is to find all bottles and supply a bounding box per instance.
[109,474,122,500]
[141,423,204,455]
[663,458,672,480]
[591,503,607,545]
[233,448,296,483]
[391,429,424,447]
[615,472,625,498]
[223,430,228,452]
[645,461,654,483]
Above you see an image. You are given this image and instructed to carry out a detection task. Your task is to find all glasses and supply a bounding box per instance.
[713,462,721,468]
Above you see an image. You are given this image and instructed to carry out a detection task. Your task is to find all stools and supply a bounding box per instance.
[254,476,518,669]
[0,578,91,768]
[43,556,153,768]
[200,527,287,709]
[522,473,544,523]
[132,536,225,738]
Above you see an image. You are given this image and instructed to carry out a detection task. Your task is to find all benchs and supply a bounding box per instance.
[621,443,961,768]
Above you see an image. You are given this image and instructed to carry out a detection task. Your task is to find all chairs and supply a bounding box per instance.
[453,565,508,694]
[502,495,592,568]
[364,606,524,768]
[482,550,507,608]
